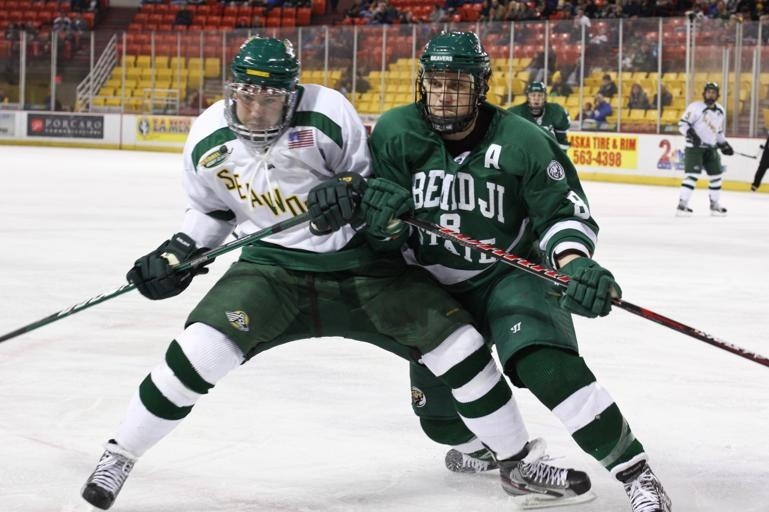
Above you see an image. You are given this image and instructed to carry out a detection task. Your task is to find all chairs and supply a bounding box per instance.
[1,0,769,141]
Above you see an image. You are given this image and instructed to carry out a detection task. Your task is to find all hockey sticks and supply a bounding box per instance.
[686,139,762,159]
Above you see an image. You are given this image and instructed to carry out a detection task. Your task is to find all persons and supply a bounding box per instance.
[674,82,732,215]
[752,134,769,190]
[356,29,670,511]
[1,1,769,136]
[504,81,570,154]
[78,36,595,508]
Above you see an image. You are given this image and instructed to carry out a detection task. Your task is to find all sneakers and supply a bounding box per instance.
[677,199,693,213]
[615,459,672,512]
[80,439,138,510]
[709,195,727,213]
[445,449,591,496]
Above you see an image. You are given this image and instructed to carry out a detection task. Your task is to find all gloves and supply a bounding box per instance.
[305,172,415,242]
[685,128,701,148]
[716,141,733,155]
[127,232,215,300]
[548,257,621,318]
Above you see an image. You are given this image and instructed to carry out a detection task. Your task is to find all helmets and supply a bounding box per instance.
[702,81,721,105]
[415,30,492,135]
[225,35,301,149]
[524,81,546,117]
